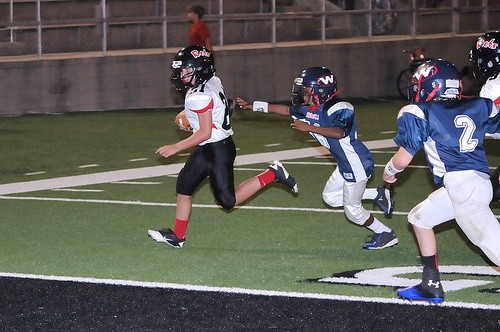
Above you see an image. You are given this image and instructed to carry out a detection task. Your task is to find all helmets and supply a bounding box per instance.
[407,60,463,102]
[172,45,214,84]
[300,67,337,106]
[470,30,500,82]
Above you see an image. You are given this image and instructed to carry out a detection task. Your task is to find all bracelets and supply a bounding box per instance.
[386,158,404,177]
[253,100,269,114]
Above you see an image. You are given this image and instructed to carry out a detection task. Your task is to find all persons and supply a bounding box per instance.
[187,6,214,52]
[147,45,300,249]
[384,58,500,304]
[470,29,500,201]
[237,67,398,250]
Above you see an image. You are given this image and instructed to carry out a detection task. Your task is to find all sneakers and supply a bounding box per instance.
[396,284,445,305]
[362,229,398,250]
[268,160,300,194]
[373,184,395,219]
[147,228,185,248]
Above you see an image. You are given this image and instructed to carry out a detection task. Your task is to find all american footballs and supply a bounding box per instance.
[175,112,193,130]
